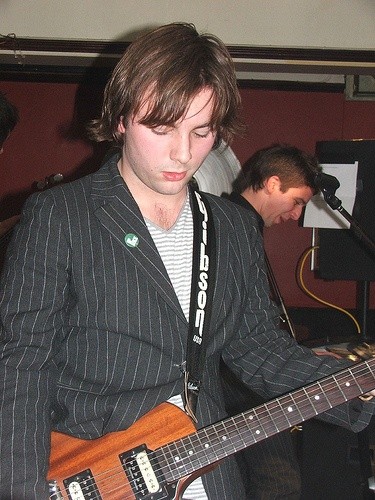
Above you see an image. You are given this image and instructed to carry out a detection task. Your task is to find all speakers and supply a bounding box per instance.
[297,417,375,500]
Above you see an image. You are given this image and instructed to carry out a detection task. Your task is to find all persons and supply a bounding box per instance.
[0,21,375,500]
[215,142,321,238]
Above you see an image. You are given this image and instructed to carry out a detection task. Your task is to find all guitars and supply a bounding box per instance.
[42,350,375,499]
